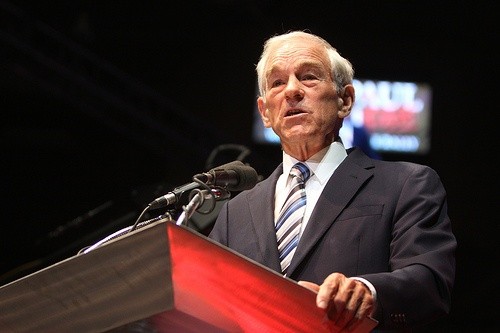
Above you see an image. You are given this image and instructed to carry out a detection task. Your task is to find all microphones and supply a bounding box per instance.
[148,160,245,211]
[203,165,258,191]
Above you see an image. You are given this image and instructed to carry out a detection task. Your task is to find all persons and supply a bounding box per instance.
[206,31,459,333]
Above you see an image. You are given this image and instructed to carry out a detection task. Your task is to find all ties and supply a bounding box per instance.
[276,162,315,278]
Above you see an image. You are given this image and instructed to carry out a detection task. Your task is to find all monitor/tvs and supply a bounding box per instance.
[263,78,432,157]
